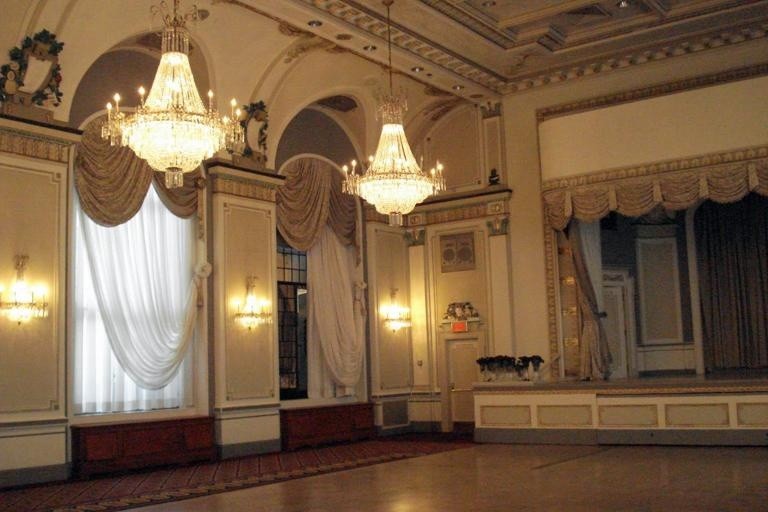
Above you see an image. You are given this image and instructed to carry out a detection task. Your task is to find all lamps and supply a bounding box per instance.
[341,0,449,227]
[0,256,50,326]
[228,274,273,331]
[379,287,412,334]
[99,1,253,192]
